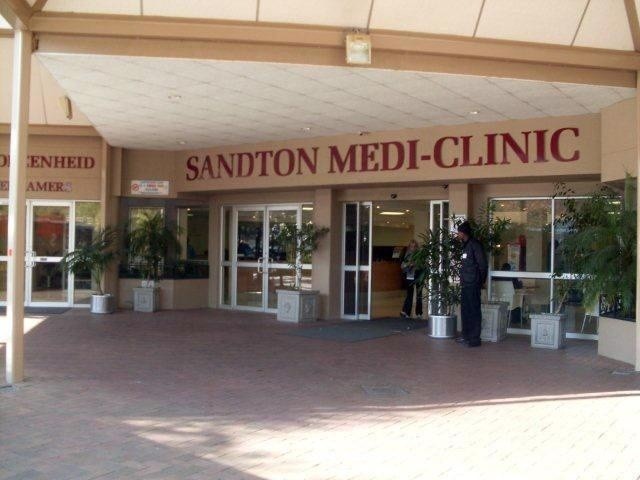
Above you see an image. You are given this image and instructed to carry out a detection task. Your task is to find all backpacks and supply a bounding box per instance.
[404,253,421,282]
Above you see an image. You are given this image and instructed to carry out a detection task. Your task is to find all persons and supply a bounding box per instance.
[398,239,425,318]
[453,221,487,346]
[497,264,526,324]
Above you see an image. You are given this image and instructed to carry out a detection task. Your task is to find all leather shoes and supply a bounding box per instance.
[455,336,481,348]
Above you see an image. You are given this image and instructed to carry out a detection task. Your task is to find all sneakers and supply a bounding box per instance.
[400,311,425,321]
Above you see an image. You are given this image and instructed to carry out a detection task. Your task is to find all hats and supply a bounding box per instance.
[457,221,471,234]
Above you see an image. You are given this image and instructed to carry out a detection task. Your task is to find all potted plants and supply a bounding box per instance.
[58,210,184,316]
[273,221,330,325]
[526,178,636,367]
[402,197,512,340]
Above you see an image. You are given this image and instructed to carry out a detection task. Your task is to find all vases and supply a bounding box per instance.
[480,299,510,343]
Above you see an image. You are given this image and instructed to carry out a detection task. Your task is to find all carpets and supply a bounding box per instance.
[287,315,428,341]
